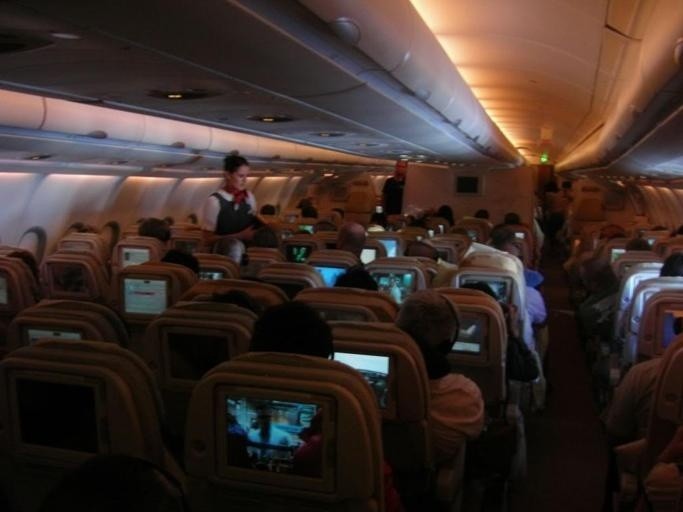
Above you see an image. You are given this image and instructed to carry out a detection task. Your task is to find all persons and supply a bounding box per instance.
[563,222,626,271]
[6,247,43,293]
[551,178,571,238]
[434,204,458,225]
[377,170,404,214]
[327,207,347,225]
[606,316,683,455]
[135,218,175,250]
[333,266,383,301]
[661,251,683,279]
[246,398,293,475]
[212,234,251,276]
[392,288,528,511]
[211,288,259,318]
[225,412,249,464]
[162,248,202,288]
[460,281,541,411]
[387,278,401,304]
[308,219,335,239]
[255,207,279,230]
[473,208,495,223]
[332,221,368,267]
[502,211,526,230]
[200,150,258,252]
[293,411,326,479]
[249,301,335,361]
[482,223,547,362]
[400,240,442,269]
[624,236,654,254]
[290,206,320,230]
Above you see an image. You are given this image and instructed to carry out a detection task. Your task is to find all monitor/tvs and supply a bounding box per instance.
[306,261,347,289]
[120,275,170,316]
[173,238,198,253]
[47,263,91,298]
[197,270,224,281]
[207,384,339,495]
[454,176,481,195]
[373,237,400,258]
[120,246,151,268]
[658,310,682,355]
[9,369,107,464]
[436,250,450,261]
[323,242,337,249]
[284,246,310,263]
[448,312,490,357]
[162,325,235,386]
[27,327,82,350]
[457,273,514,305]
[297,224,314,234]
[370,269,416,305]
[332,352,392,414]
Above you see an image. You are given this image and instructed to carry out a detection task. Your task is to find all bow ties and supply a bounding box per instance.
[232,188,250,204]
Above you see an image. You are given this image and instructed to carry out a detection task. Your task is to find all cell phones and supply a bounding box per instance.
[375,206,383,213]
